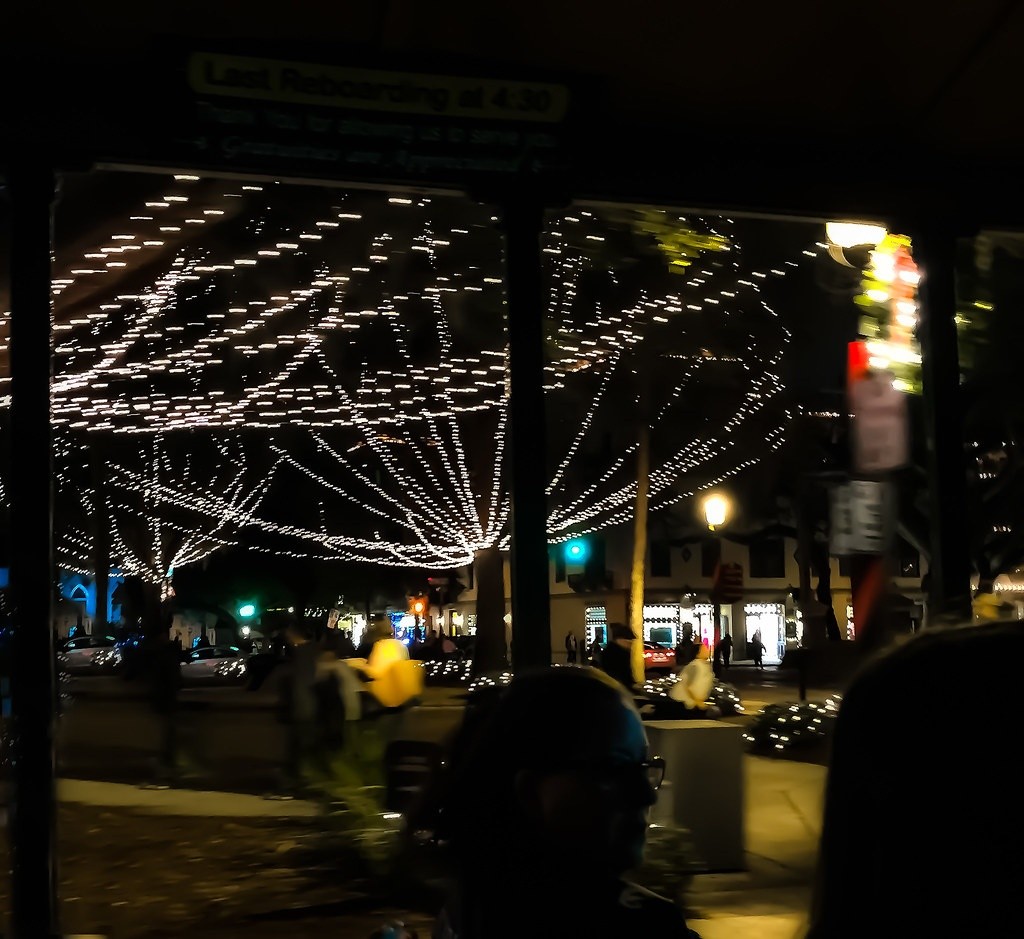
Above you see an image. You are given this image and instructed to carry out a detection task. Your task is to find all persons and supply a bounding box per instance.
[193,633,210,648]
[719,633,734,670]
[803,617,1024,939]
[71,624,86,637]
[402,623,445,642]
[750,634,766,670]
[588,638,601,656]
[345,630,353,650]
[434,663,707,939]
[565,630,577,662]
[173,632,182,650]
[674,622,698,665]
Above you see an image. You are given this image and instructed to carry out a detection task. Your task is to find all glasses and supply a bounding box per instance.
[634,754,666,785]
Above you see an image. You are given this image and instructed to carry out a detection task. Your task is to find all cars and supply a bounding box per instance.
[644,641,676,676]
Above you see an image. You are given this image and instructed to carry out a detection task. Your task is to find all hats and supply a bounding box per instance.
[674,638,699,664]
[608,621,637,640]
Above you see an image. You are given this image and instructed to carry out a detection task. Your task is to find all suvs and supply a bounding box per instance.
[56,636,125,673]
[179,647,249,685]
